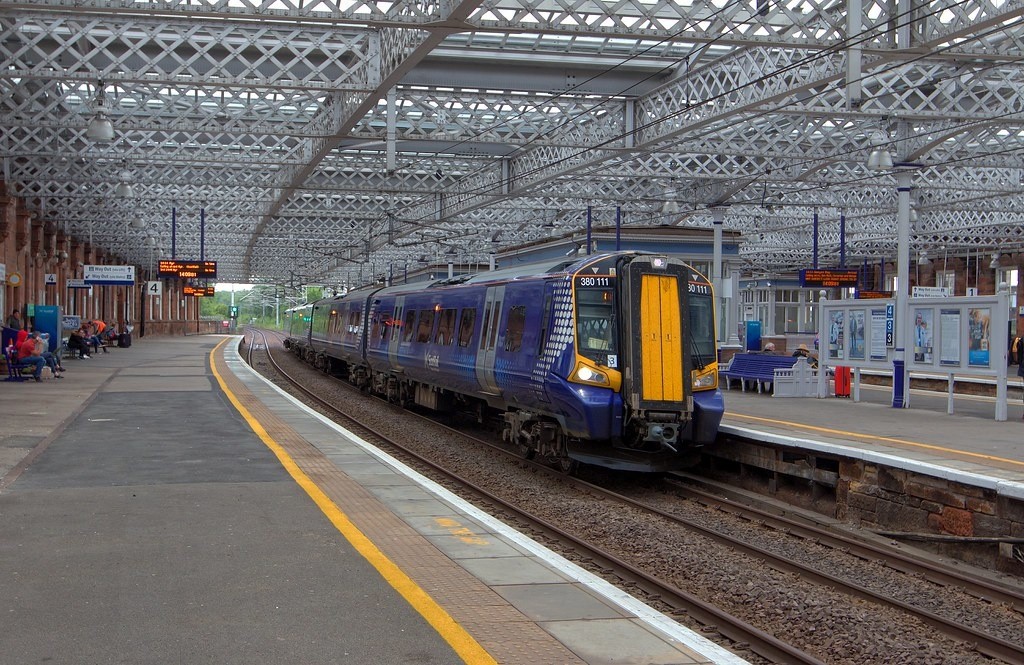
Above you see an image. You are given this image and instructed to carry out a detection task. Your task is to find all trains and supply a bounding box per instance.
[283,256,726,477]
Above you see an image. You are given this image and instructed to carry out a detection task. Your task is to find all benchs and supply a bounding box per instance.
[106,331,118,348]
[3,347,34,382]
[62,337,79,360]
[718,353,798,394]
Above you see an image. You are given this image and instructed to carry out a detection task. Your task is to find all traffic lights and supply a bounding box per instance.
[230,306,238,319]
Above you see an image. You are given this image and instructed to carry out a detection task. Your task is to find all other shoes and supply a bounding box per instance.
[78,345,110,360]
[56,366,65,372]
[54,375,64,379]
[33,374,42,382]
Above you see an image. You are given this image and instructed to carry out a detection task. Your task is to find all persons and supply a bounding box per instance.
[15,330,66,383]
[970,311,990,351]
[68,320,107,359]
[849,314,857,349]
[106,321,121,346]
[6,309,20,329]
[793,344,810,358]
[915,313,928,361]
[830,314,839,356]
[763,343,776,353]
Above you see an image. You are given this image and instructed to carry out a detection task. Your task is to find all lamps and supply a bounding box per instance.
[766,205,774,214]
[918,255,929,265]
[661,201,679,214]
[747,232,763,243]
[909,208,918,222]
[867,148,893,170]
[566,248,577,257]
[131,217,145,228]
[989,260,1001,268]
[551,228,561,237]
[86,100,115,143]
[144,237,155,246]
[482,242,492,252]
[115,181,135,199]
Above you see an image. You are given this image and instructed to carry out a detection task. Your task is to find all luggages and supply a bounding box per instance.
[118,322,131,348]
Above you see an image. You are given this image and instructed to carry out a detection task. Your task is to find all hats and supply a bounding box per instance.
[13,309,19,315]
[796,344,810,353]
